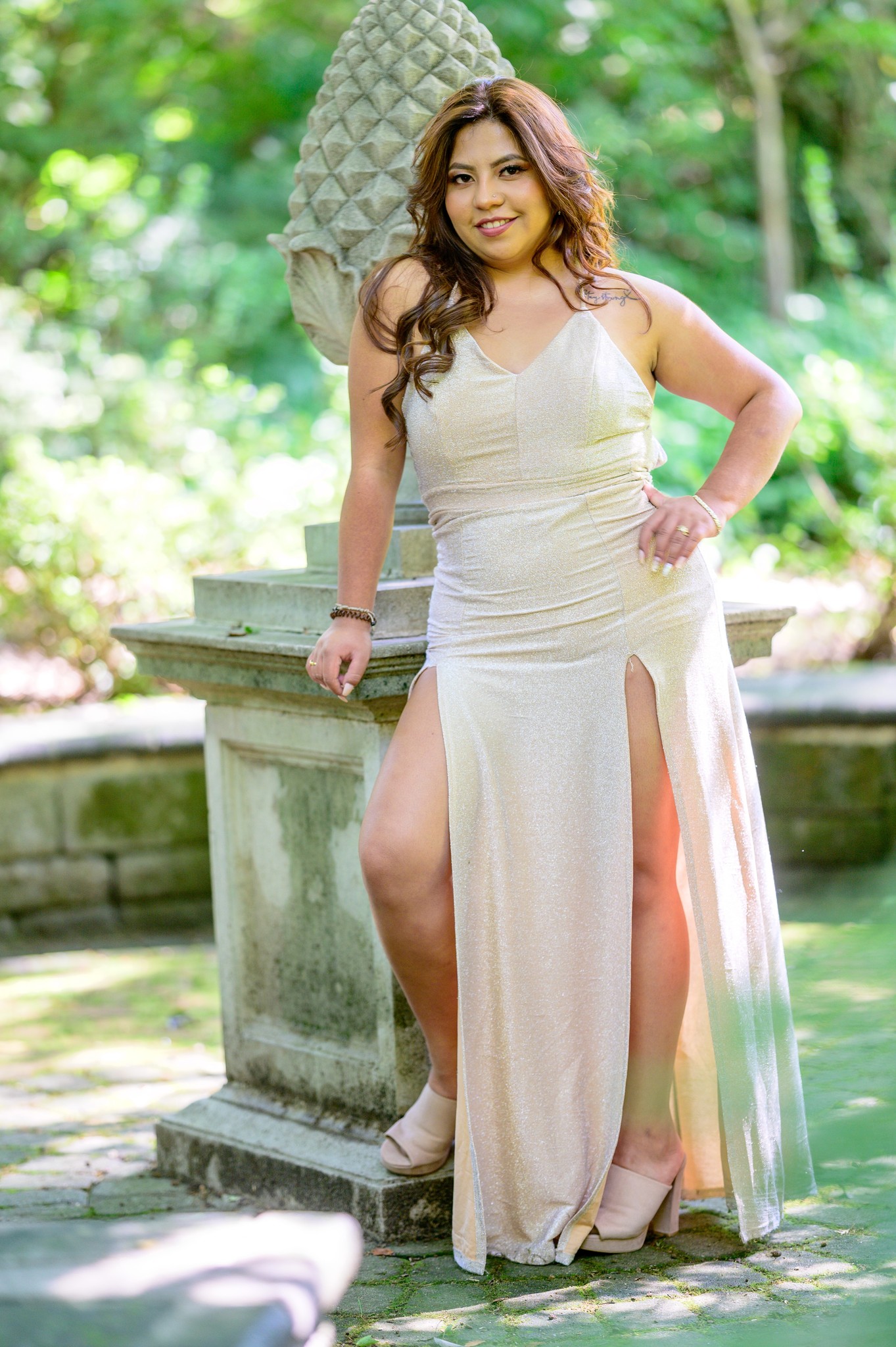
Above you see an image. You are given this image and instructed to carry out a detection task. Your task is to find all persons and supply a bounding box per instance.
[306,79,822,1276]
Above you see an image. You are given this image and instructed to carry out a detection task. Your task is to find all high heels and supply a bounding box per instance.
[380,1083,456,1175]
[579,1153,687,1253]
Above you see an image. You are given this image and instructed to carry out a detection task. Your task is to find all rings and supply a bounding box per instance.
[309,659,316,666]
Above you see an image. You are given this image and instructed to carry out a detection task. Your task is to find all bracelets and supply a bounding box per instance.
[676,525,690,537]
[330,610,377,626]
[692,495,722,536]
[332,603,375,623]
